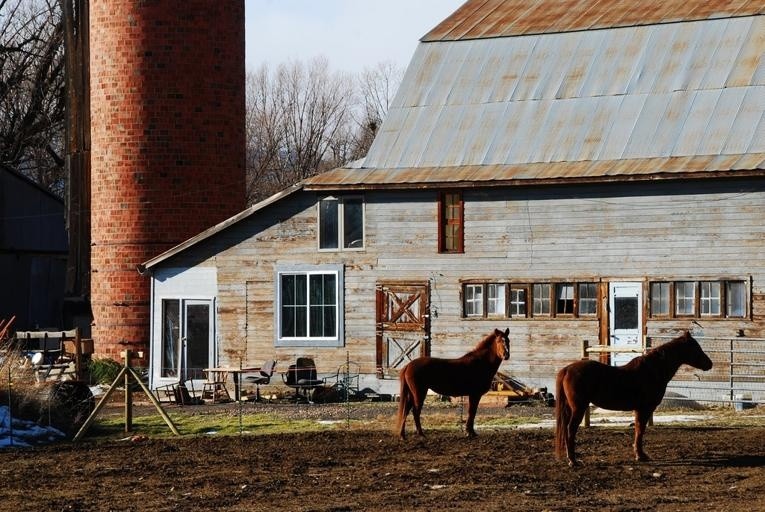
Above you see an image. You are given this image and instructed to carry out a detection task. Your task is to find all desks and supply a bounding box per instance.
[203,366,261,404]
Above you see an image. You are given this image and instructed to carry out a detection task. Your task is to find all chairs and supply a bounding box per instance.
[244,357,361,404]
[155,365,231,406]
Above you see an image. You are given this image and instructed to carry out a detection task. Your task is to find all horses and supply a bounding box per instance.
[551,329,714,469]
[395,326,512,444]
